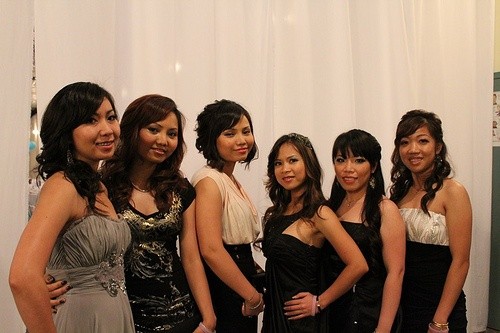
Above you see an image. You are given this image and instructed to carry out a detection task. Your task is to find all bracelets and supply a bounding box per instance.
[428,319,450,333]
[245,292,263,310]
[198,322,217,333]
[312,296,322,317]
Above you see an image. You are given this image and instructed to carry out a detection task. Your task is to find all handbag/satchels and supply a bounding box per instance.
[214,271,267,315]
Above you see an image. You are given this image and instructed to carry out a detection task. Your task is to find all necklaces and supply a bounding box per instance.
[129,179,154,192]
[344,193,365,207]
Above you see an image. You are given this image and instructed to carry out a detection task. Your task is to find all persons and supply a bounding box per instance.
[387,109,474,333]
[325,128,406,333]
[254,132,370,333]
[8,80,137,332]
[187,99,268,333]
[41,94,218,333]
